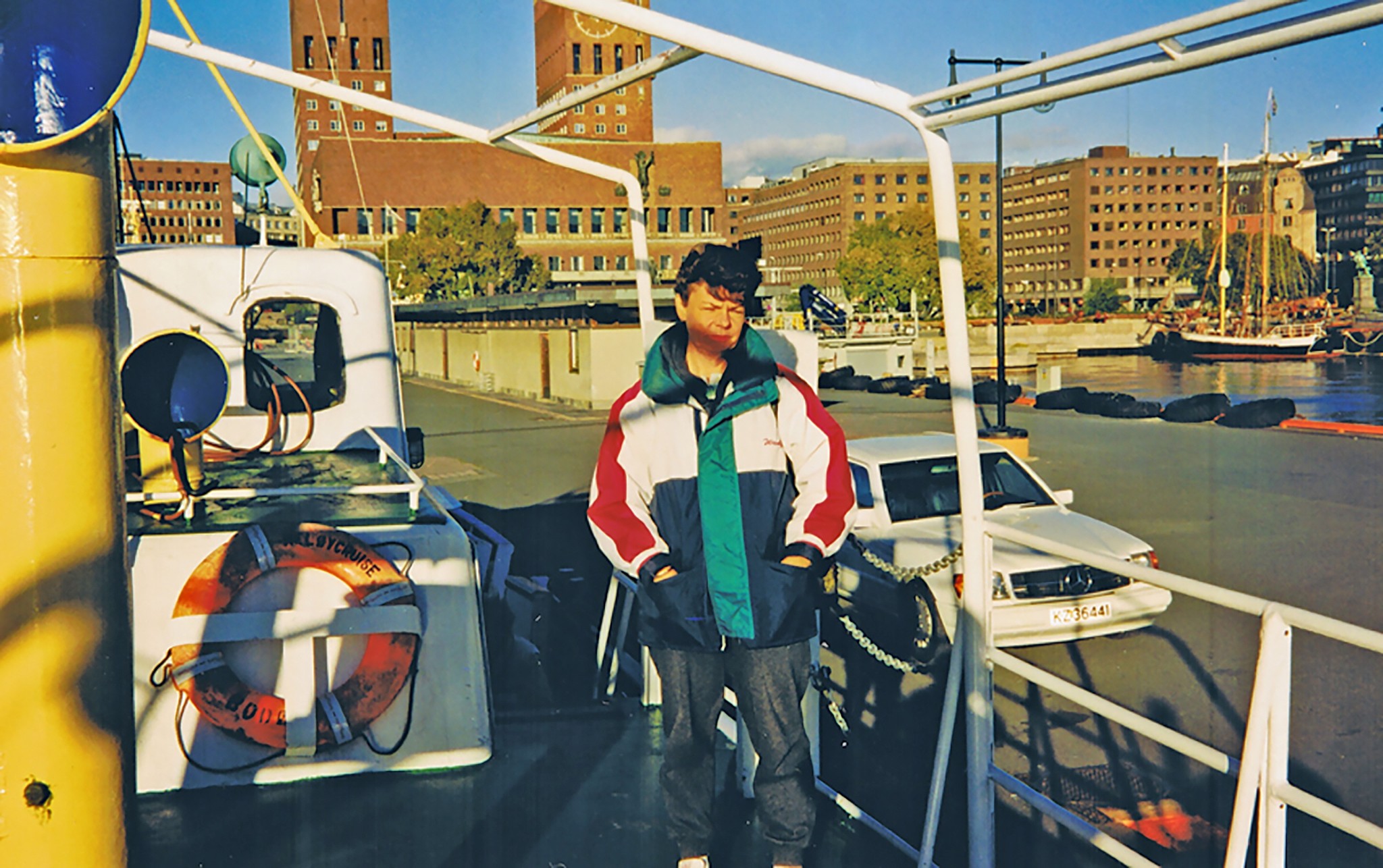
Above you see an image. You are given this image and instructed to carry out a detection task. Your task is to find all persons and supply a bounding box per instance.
[586,244,859,868]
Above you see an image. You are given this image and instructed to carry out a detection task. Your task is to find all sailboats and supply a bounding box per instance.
[1147,85,1332,361]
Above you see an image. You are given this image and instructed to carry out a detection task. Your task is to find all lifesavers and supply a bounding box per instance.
[164,517,427,754]
[819,364,1022,403]
[1224,398,1297,427]
[1164,392,1230,423]
[473,350,480,371]
[848,322,864,338]
[1151,330,1181,361]
[1035,386,1163,418]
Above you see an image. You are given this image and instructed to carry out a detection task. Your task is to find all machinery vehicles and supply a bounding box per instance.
[772,284,849,339]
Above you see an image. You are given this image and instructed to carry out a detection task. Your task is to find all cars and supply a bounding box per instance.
[829,431,1173,672]
